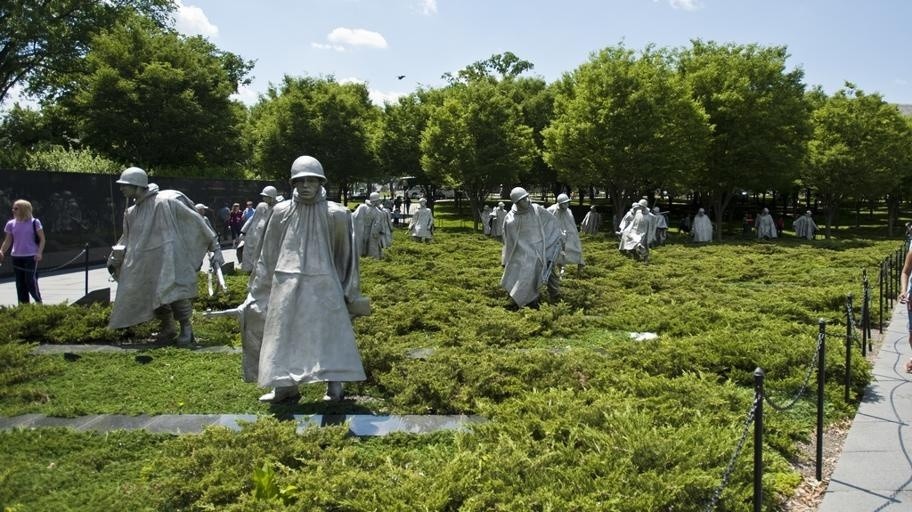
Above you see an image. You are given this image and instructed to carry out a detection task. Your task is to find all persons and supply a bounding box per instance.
[195,201,256,242]
[108,167,225,347]
[742,211,754,232]
[792,210,820,240]
[408,198,433,243]
[754,207,778,241]
[690,206,715,244]
[684,212,692,234]
[678,215,687,235]
[779,216,785,240]
[236,151,363,415]
[619,199,668,262]
[0,197,50,308]
[744,212,754,239]
[353,191,393,259]
[500,186,585,309]
[898,236,912,376]
[581,205,600,233]
[481,202,507,235]
[794,211,800,218]
[239,185,286,272]
[383,193,412,223]
